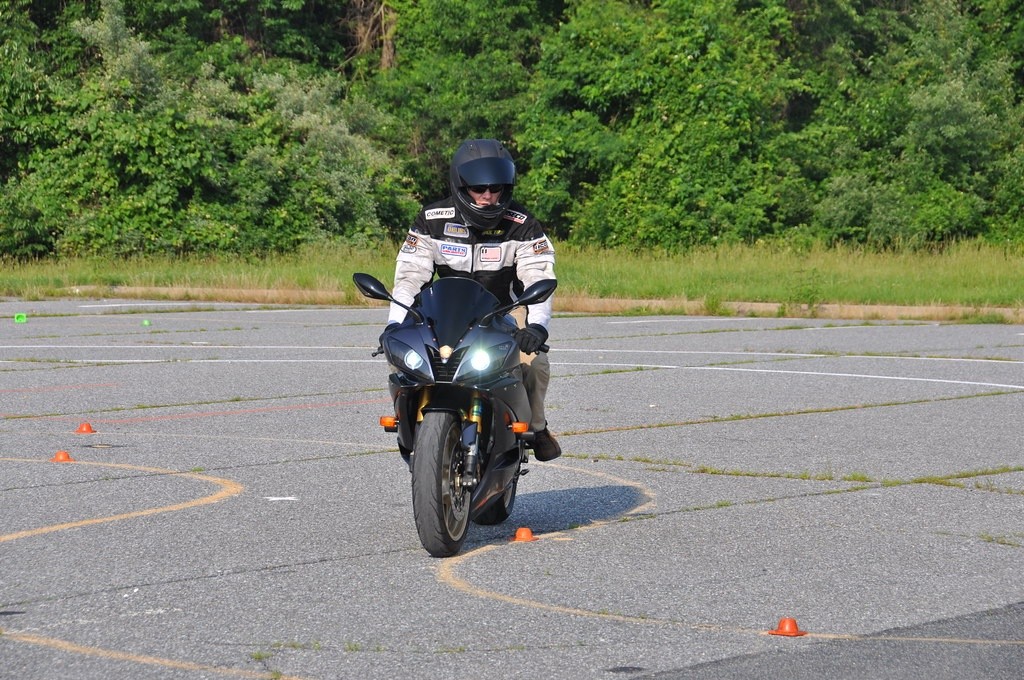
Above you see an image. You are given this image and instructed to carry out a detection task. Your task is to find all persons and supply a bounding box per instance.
[379,138,562,461]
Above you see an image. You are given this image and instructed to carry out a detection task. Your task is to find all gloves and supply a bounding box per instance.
[384,323,400,330]
[515,323,548,355]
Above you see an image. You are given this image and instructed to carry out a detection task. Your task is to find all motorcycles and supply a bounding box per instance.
[350,272,561,558]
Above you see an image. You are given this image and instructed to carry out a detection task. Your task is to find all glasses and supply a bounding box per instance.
[467,184,504,194]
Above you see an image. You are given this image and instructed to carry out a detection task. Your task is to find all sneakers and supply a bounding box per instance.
[526,428,561,461]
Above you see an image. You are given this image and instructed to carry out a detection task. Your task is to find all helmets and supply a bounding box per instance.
[449,139,517,230]
